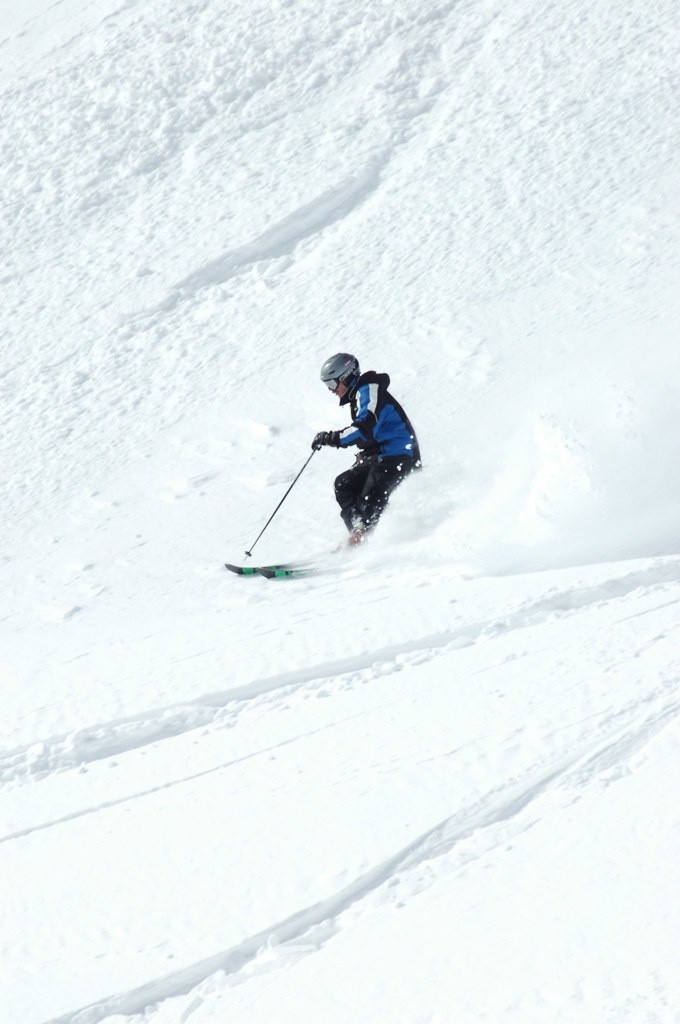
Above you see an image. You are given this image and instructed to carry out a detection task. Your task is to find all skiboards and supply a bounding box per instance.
[224,548,365,579]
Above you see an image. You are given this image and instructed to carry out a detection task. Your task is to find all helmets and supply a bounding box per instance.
[320,353,360,381]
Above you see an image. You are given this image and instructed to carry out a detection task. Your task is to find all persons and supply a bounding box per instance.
[311,353,423,552]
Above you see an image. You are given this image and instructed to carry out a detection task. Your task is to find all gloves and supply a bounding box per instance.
[311,430,340,450]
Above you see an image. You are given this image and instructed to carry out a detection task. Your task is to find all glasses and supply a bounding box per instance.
[322,378,339,393]
